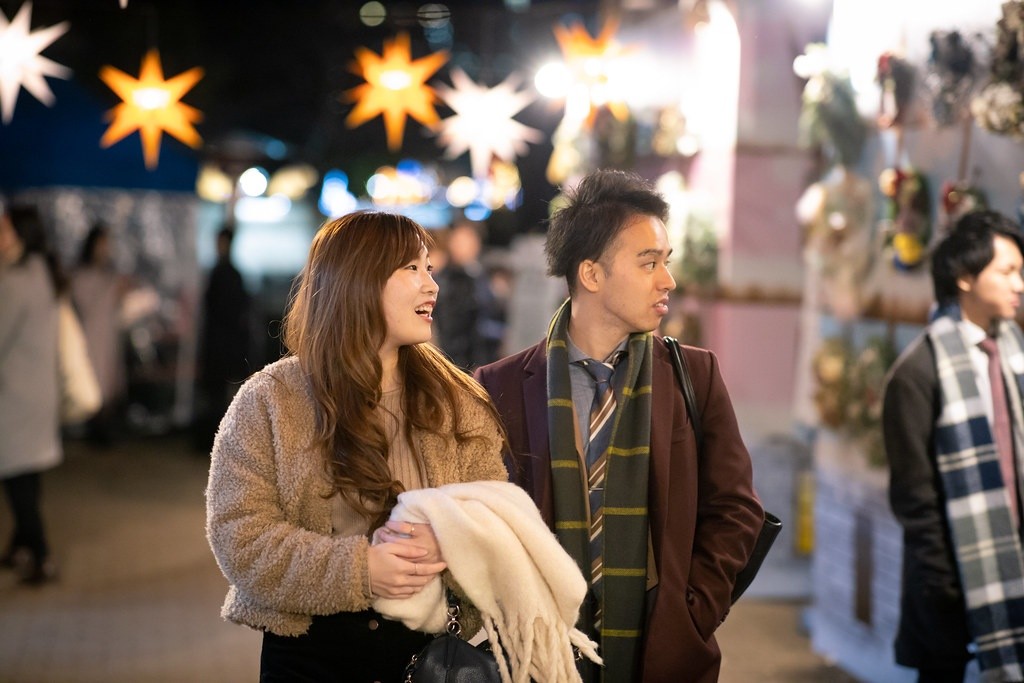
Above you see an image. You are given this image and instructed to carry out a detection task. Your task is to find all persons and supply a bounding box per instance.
[467,167,767,683]
[0,201,257,588]
[204,209,520,682]
[880,211,1024,683]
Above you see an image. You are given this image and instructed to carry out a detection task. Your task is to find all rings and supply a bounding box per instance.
[408,521,417,538]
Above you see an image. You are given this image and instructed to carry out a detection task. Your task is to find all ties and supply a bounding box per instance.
[975,336,1020,532]
[575,358,618,634]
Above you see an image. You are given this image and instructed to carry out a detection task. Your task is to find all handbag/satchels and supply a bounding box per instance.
[56,291,103,430]
[401,590,513,682]
[663,334,782,606]
